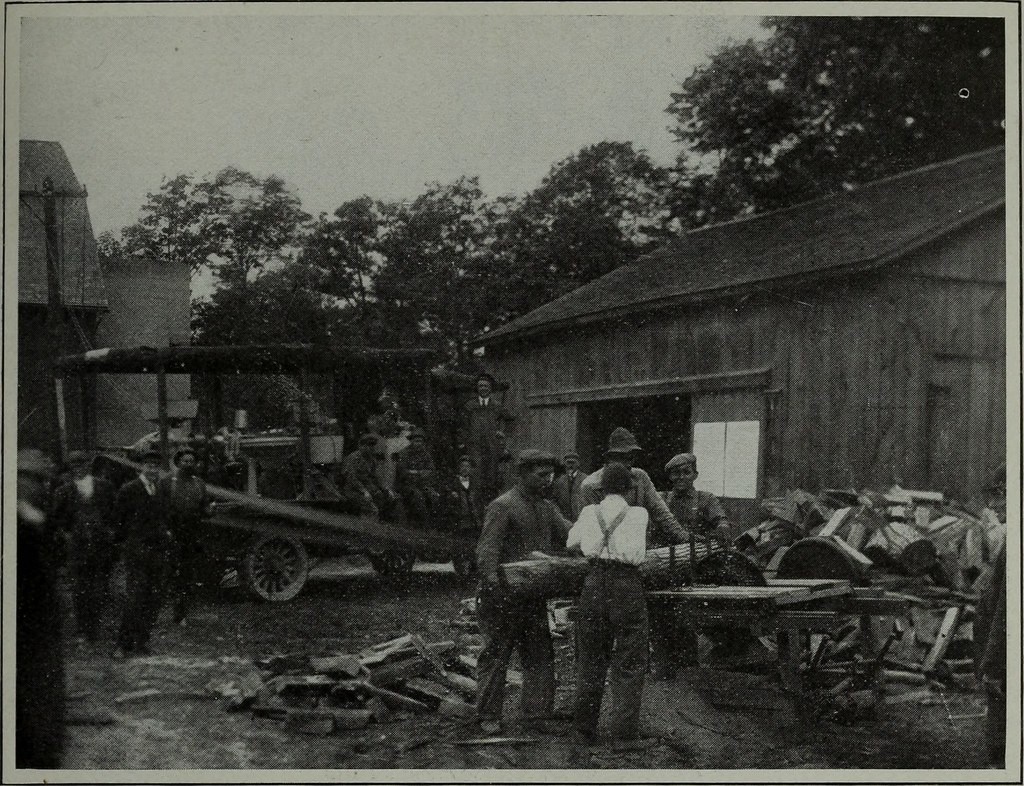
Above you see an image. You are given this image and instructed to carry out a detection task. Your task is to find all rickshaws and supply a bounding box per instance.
[88,455,481,604]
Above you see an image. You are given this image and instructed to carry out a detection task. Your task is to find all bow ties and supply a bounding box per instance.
[459,476,469,482]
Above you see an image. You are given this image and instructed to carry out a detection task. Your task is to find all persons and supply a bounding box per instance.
[958,461,1006,769]
[17,374,733,770]
[565,462,658,753]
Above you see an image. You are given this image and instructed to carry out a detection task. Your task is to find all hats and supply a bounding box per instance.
[142,450,162,463]
[516,450,554,465]
[173,447,201,468]
[600,461,633,492]
[457,455,473,464]
[472,372,500,394]
[66,451,92,470]
[984,463,1006,492]
[665,453,696,469]
[406,428,427,441]
[601,427,643,457]
[564,453,579,463]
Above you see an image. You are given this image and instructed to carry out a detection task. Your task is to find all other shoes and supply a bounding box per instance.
[613,737,658,754]
[480,719,502,735]
[530,718,571,735]
[571,730,602,745]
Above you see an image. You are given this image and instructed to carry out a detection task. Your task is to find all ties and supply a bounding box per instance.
[149,484,155,495]
[481,400,486,412]
